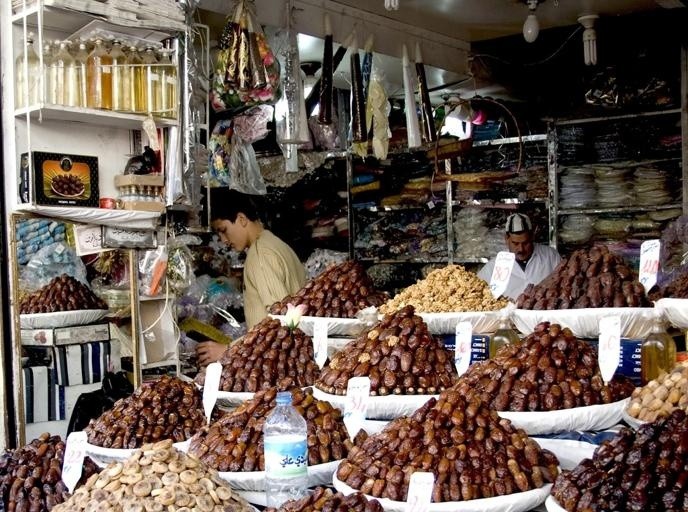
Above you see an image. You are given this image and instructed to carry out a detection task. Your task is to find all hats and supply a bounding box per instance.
[504,212,535,233]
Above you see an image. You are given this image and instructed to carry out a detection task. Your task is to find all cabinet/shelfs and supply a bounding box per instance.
[347,107,687,300]
[0,1,191,220]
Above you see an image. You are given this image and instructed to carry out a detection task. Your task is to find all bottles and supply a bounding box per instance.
[263,392,310,511]
[641,316,676,386]
[14,34,179,118]
[119,189,162,202]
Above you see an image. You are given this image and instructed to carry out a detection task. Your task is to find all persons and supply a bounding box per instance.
[476,210,561,301]
[194,195,305,366]
